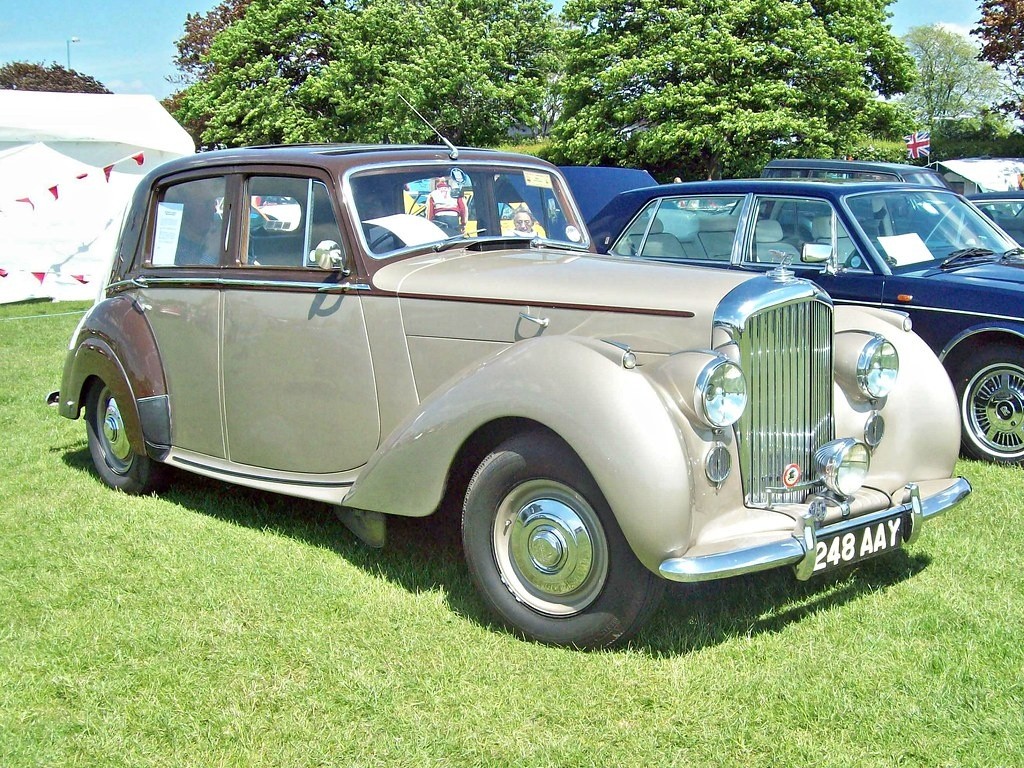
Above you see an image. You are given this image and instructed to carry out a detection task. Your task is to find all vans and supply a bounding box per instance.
[755,157,984,248]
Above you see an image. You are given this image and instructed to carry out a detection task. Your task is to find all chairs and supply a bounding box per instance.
[692,215,739,258]
[624,215,689,258]
[358,189,395,254]
[810,215,856,264]
[998,216,1024,246]
[755,219,803,263]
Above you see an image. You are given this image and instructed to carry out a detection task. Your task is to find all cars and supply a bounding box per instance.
[479,166,1024,466]
[960,190,1024,246]
[46,143,973,653]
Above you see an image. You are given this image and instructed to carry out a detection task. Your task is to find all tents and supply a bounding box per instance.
[0,93,196,307]
[921,153,1024,193]
[466,166,659,246]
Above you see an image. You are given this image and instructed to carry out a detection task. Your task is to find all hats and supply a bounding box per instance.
[435,181,449,188]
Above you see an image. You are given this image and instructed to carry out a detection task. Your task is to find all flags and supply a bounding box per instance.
[903,129,930,160]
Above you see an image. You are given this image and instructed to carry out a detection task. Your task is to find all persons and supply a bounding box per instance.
[505,202,543,239]
[425,178,467,236]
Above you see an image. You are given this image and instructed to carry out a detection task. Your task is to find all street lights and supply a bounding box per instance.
[67,37,79,72]
